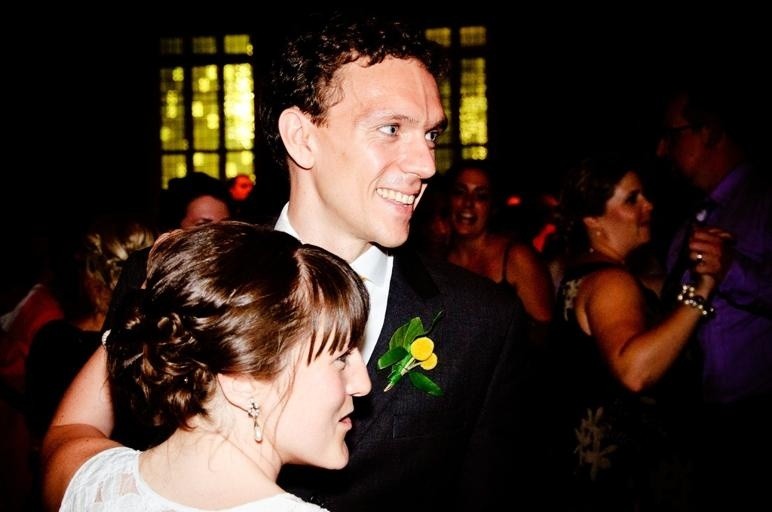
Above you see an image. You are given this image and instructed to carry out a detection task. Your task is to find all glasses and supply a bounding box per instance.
[660,122,704,141]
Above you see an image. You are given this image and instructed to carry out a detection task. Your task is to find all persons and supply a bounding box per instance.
[246,12,529,512]
[42,221,372,512]
